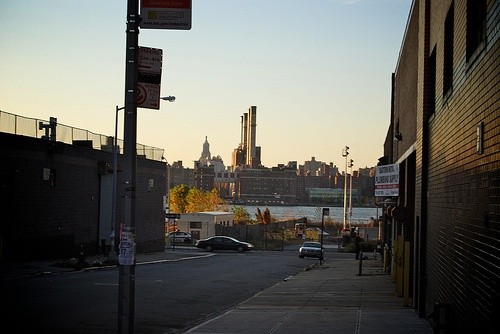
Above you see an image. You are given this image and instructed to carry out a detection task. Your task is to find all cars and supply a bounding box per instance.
[164,230,192,245]
[299,242,326,260]
[195,236,255,253]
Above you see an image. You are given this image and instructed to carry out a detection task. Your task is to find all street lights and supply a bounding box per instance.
[342,145,349,230]
[105,96,176,264]
[347,159,354,231]
